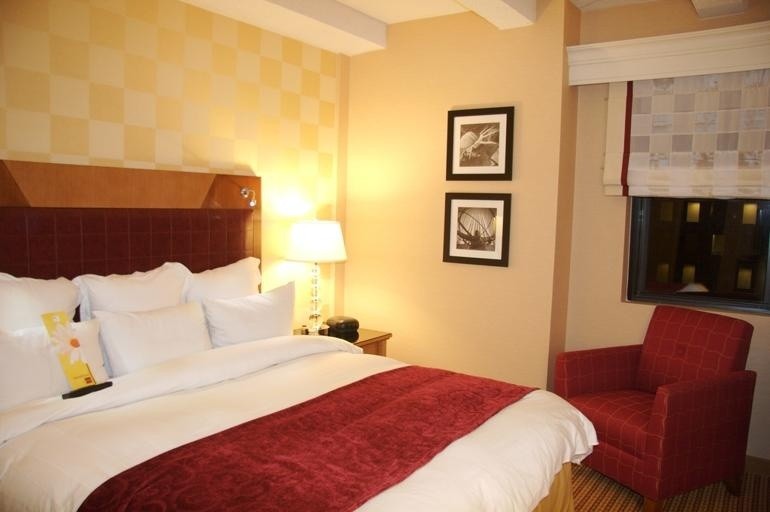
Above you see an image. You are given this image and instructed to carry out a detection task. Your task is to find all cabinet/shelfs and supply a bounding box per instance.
[332,327,392,356]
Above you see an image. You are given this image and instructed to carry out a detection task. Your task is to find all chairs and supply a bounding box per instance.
[554,305,757,512]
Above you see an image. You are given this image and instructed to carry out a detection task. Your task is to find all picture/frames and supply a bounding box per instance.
[442,192,512,267]
[446,105,515,181]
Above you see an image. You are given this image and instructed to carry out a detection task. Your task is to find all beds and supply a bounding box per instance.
[0,158,601,511]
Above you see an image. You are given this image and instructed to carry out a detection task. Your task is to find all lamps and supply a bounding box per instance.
[285,219,349,332]
[241,186,257,208]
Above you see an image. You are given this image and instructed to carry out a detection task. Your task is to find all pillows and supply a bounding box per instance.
[0,317,111,414]
[0,273,83,329]
[181,256,262,307]
[73,260,191,380]
[92,299,212,378]
[201,281,297,348]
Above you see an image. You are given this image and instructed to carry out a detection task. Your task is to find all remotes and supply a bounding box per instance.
[62,382,112,399]
